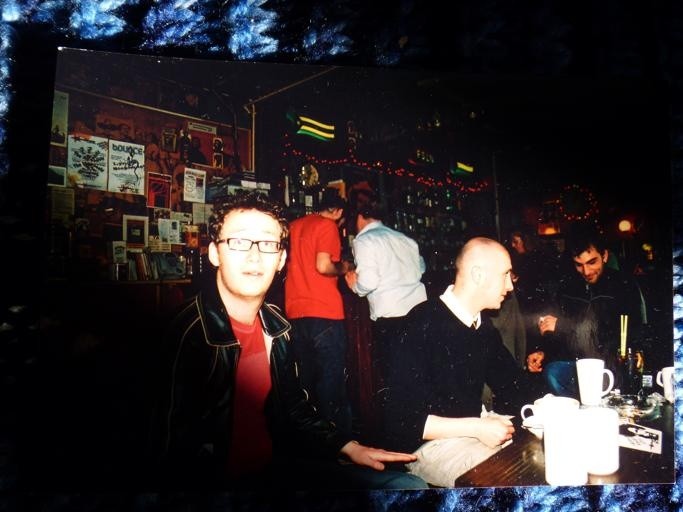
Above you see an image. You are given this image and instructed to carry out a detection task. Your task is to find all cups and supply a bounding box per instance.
[655,366,673,403]
[543,415,588,486]
[519,395,580,424]
[576,357,615,407]
[579,408,621,473]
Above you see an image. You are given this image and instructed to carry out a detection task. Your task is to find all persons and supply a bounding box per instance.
[283,196,354,437]
[551,235,649,357]
[81,72,211,166]
[342,206,428,432]
[140,190,426,489]
[370,236,542,450]
[508,225,560,309]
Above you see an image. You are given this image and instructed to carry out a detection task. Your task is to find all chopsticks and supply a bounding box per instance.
[620,314,628,357]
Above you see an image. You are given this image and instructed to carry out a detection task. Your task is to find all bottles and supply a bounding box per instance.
[618,347,642,396]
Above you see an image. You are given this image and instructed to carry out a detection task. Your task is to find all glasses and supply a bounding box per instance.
[215,237,284,254]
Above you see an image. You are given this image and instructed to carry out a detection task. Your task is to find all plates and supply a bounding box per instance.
[521,419,543,439]
